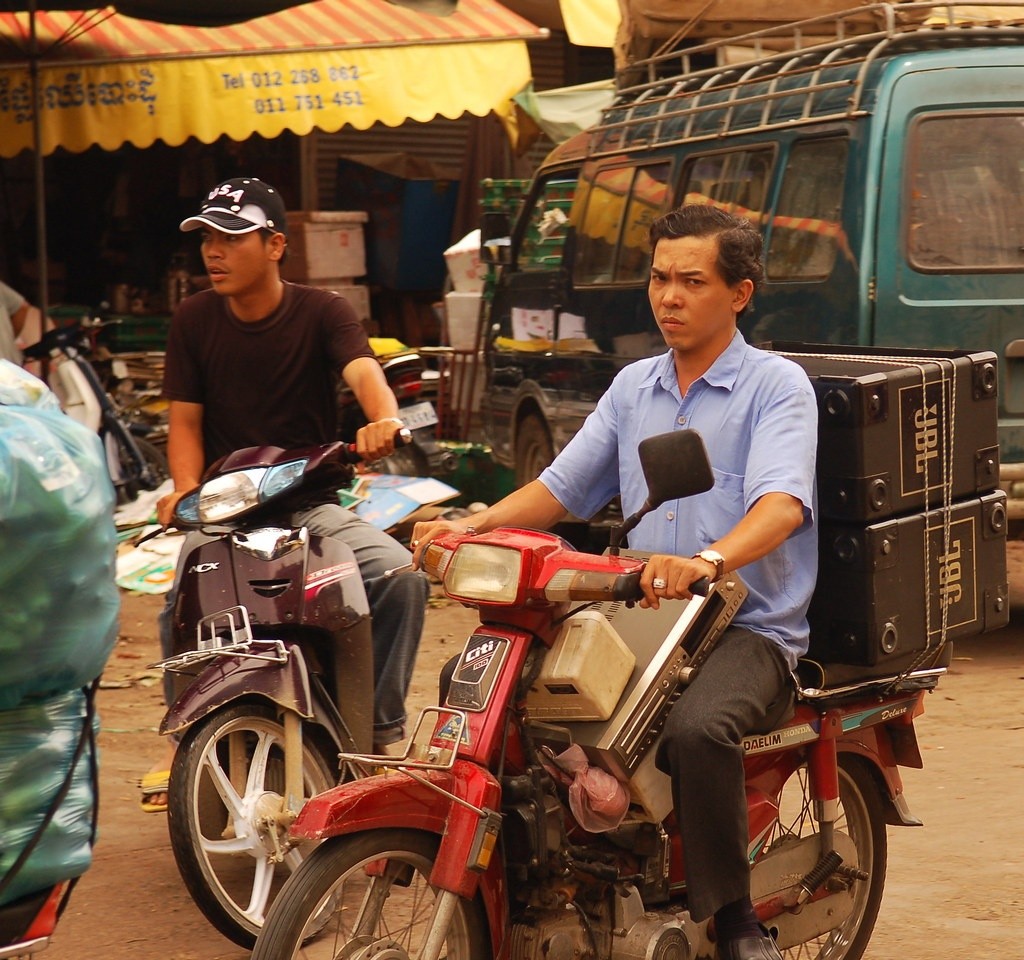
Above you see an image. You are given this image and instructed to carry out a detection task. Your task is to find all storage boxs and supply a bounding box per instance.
[284,211,505,415]
[526,612,638,723]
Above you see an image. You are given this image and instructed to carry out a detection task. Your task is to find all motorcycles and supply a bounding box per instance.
[15,312,169,506]
[335,333,456,480]
[134,432,417,949]
[248,428,948,960]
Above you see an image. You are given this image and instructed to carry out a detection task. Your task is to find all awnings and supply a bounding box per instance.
[0,0,547,160]
[566,152,866,282]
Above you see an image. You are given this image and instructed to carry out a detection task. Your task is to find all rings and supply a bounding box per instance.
[412,541,419,546]
[653,579,666,588]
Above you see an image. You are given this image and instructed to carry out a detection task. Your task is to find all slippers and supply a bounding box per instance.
[141,771,172,812]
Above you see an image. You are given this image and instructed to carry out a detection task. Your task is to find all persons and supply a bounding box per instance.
[0,273,29,366]
[141,177,431,812]
[409,203,818,960]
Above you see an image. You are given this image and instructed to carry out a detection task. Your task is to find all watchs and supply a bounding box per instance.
[692,550,725,583]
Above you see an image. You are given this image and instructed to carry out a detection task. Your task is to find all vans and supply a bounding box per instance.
[478,1,1023,542]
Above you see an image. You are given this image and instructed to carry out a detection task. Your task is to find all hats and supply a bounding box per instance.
[180,177,288,241]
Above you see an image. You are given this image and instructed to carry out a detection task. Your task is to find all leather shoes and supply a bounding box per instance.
[714,922,782,960]
